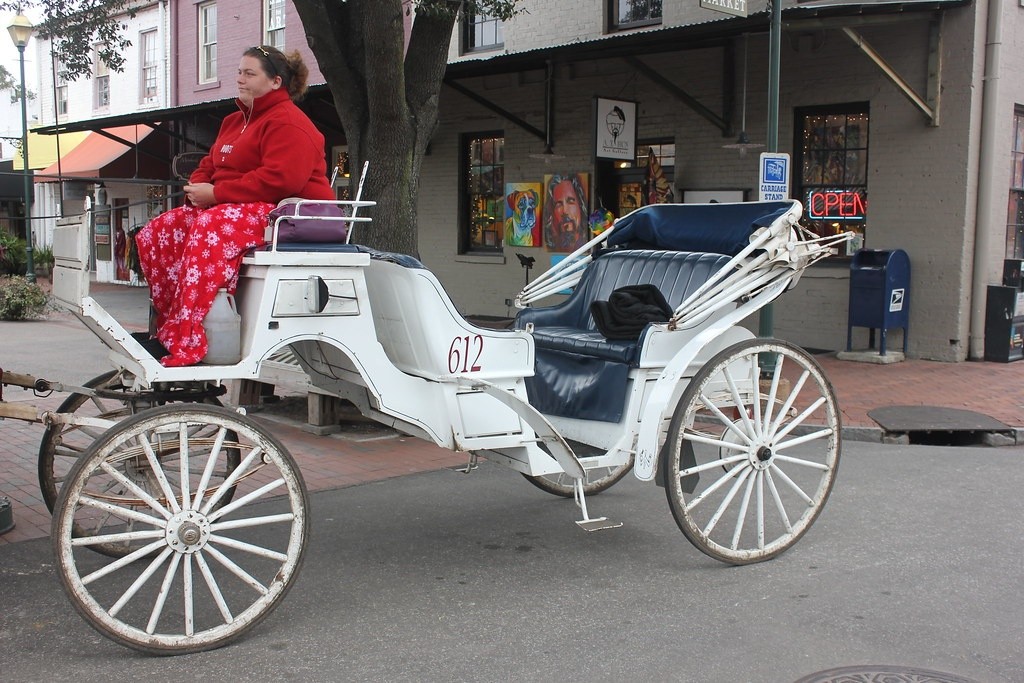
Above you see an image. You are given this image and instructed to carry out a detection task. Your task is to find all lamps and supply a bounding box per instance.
[721,32,766,158]
[94,182,111,190]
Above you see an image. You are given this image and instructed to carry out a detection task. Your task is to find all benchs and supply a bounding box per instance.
[352,242,536,380]
[514,249,731,364]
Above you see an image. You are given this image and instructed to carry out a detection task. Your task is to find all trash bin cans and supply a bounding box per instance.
[844,247,912,360]
[986,284,1024,363]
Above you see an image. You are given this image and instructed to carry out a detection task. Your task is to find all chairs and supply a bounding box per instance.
[245,160,379,250]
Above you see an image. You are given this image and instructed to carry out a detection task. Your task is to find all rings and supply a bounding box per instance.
[192,200,194,204]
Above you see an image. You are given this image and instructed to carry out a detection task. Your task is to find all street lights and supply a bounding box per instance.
[6,8,37,286]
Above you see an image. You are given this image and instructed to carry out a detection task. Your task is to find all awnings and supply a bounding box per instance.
[28,0,943,165]
[13,121,172,184]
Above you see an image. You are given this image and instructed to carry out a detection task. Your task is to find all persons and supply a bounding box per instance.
[130,46,338,367]
